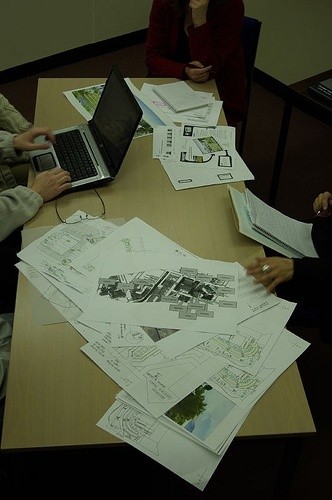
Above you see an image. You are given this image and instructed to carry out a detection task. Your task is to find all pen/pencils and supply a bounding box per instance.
[315,197,331,216]
[185,63,219,75]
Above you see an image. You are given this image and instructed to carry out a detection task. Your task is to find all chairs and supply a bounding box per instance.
[141,16,262,161]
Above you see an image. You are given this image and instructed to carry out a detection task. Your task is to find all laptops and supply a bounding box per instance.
[27,65,143,193]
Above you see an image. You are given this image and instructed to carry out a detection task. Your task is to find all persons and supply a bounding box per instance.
[0,92,73,247]
[143,0,249,126]
[242,183,332,330]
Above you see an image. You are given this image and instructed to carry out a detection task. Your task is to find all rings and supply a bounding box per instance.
[263,263,271,272]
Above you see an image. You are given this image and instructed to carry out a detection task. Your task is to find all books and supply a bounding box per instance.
[153,81,208,113]
[227,184,319,258]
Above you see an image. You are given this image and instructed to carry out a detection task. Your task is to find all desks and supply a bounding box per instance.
[0,77,318,500]
[268,68,332,207]
